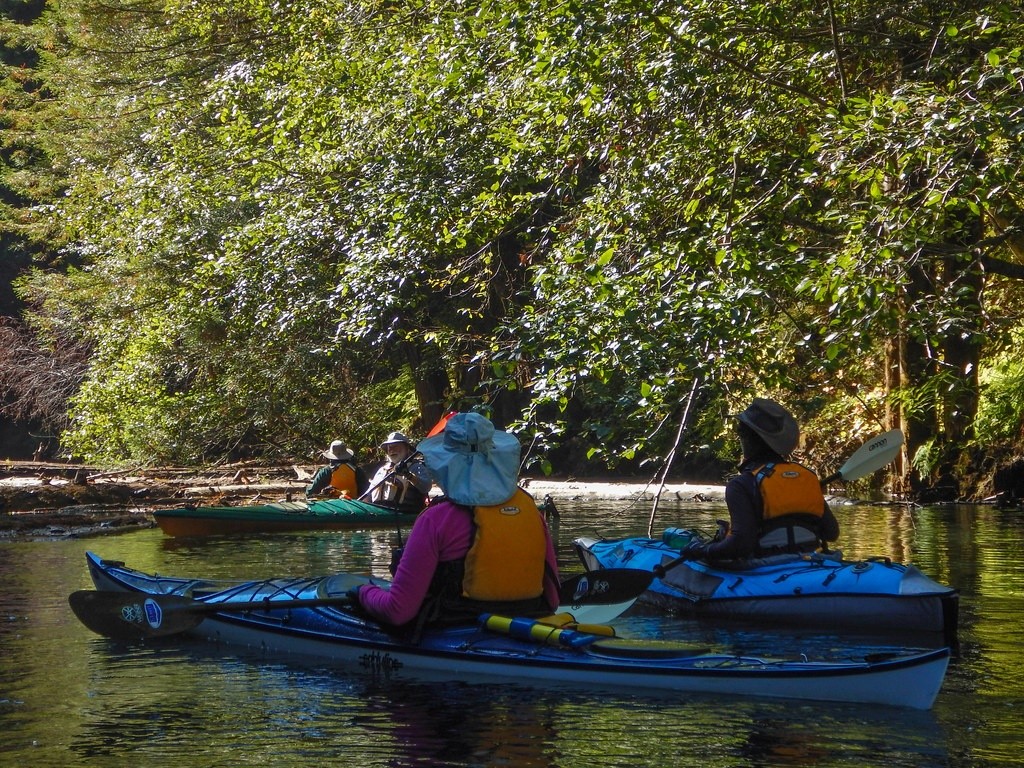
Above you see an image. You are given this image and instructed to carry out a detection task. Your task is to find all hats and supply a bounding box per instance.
[416,411,522,506]
[322,440,354,460]
[380,432,411,452]
[727,398,799,461]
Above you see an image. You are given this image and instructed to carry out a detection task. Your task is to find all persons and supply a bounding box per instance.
[681,398,840,567]
[306,440,369,501]
[346,411,560,627]
[363,432,433,509]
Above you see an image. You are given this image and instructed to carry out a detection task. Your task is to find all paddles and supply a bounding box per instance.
[559,428,904,628]
[355,417,449,501]
[68,573,352,640]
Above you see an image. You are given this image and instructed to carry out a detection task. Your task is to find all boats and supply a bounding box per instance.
[572,535,961,635]
[152,494,559,538]
[85,549,951,712]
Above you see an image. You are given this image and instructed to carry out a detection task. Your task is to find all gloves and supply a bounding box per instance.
[346,585,364,609]
[680,537,705,562]
[394,459,408,477]
